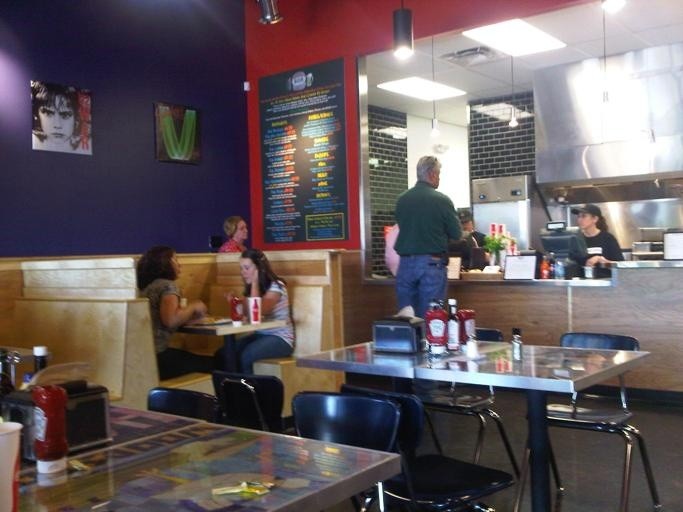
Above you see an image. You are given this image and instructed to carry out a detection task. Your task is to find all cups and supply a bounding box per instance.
[247,296,263,325]
[485,223,518,270]
[0,420,23,512]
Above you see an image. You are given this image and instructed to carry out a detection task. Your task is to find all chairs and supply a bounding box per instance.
[211,363,288,432]
[347,379,513,510]
[515,326,660,512]
[213,279,347,416]
[0,291,215,408]
[148,382,218,422]
[437,325,517,473]
[288,387,401,454]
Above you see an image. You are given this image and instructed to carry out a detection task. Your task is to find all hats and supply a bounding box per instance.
[457,210,472,223]
[571,205,601,217]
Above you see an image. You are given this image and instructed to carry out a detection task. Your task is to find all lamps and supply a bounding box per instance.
[393,0,413,57]
[257,0,284,25]
[508,56,518,128]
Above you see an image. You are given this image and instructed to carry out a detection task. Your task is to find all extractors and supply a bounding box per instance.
[532,89,683,206]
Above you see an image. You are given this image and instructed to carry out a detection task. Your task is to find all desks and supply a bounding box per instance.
[291,330,650,510]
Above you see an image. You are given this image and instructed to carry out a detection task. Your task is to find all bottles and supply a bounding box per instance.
[32,345,47,374]
[35,473,70,512]
[511,334,523,362]
[538,252,566,279]
[511,360,522,375]
[0,348,8,375]
[5,349,24,393]
[17,373,33,393]
[29,382,69,475]
[229,297,243,328]
[427,357,478,373]
[424,297,478,353]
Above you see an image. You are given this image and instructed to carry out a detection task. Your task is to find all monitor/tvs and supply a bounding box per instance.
[539,226,588,258]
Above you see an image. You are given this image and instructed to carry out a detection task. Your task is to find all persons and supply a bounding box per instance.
[30,80,92,155]
[457,211,490,272]
[391,155,462,320]
[210,249,295,399]
[217,216,249,253]
[135,245,208,382]
[564,204,625,279]
